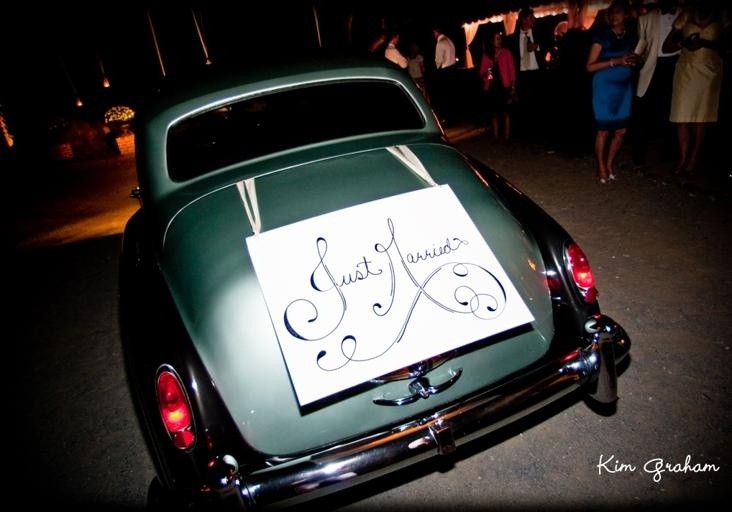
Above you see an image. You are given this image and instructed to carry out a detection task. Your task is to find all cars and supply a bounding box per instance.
[116,57,636,511]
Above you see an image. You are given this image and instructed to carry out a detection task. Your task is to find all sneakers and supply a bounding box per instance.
[674,156,688,174]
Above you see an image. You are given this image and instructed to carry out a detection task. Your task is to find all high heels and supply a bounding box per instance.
[595,173,618,184]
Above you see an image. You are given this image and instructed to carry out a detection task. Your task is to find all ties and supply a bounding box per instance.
[523,31,529,68]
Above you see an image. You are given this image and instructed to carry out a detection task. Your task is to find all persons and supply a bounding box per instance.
[368,2,730,185]
